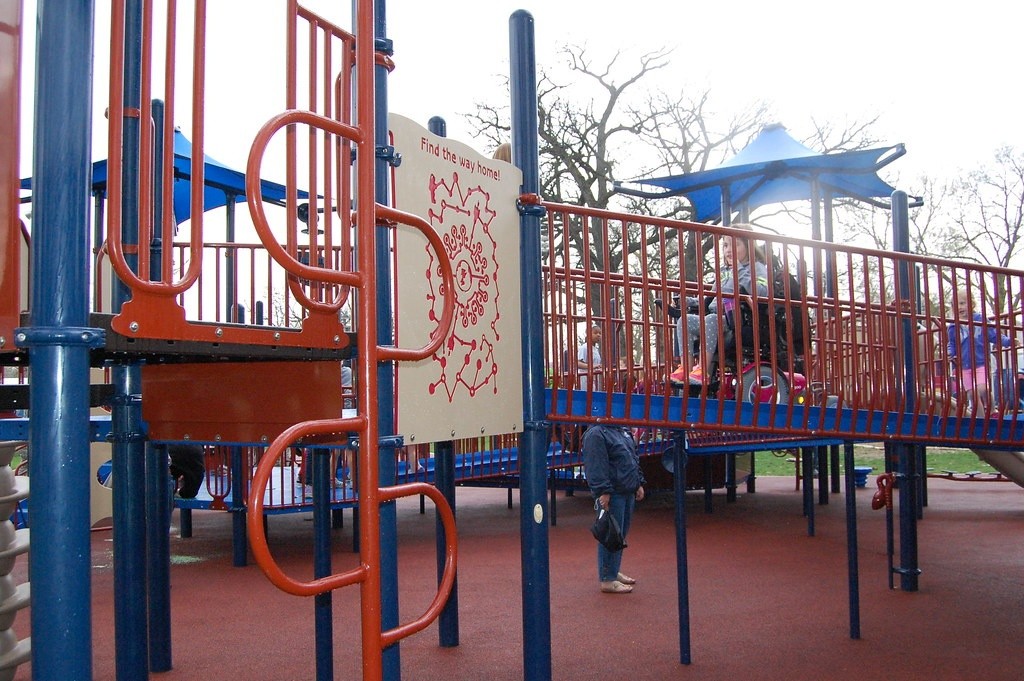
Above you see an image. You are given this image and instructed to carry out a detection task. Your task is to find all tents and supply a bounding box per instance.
[626,123,924,319]
[13,128,334,323]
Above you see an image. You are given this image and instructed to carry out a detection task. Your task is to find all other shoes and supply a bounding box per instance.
[689,368,712,386]
[670,366,692,385]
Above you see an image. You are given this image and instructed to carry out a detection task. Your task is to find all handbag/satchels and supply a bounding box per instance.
[591,511,628,553]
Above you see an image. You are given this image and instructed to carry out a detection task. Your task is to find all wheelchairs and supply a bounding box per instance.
[654,246,813,409]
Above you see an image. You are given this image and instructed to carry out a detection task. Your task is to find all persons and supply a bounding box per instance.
[662,222,768,383]
[579,423,645,593]
[330,364,353,486]
[297,448,312,498]
[169,445,206,538]
[578,324,603,389]
[937,288,1019,418]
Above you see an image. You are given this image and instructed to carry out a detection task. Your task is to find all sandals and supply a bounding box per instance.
[599,580,633,594]
[616,572,636,584]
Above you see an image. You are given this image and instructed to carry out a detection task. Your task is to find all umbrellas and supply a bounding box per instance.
[588,497,628,552]
